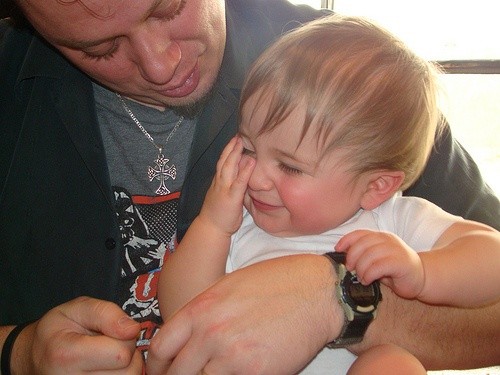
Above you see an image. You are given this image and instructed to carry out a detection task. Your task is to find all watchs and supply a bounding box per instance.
[322,252,382,349]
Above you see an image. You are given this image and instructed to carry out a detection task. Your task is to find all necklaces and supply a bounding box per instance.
[116,92,186,195]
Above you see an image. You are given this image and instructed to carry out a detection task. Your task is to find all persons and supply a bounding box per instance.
[0,0,500,375]
[157,14,500,375]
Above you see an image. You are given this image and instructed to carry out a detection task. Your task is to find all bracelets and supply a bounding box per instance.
[0,321,33,375]
